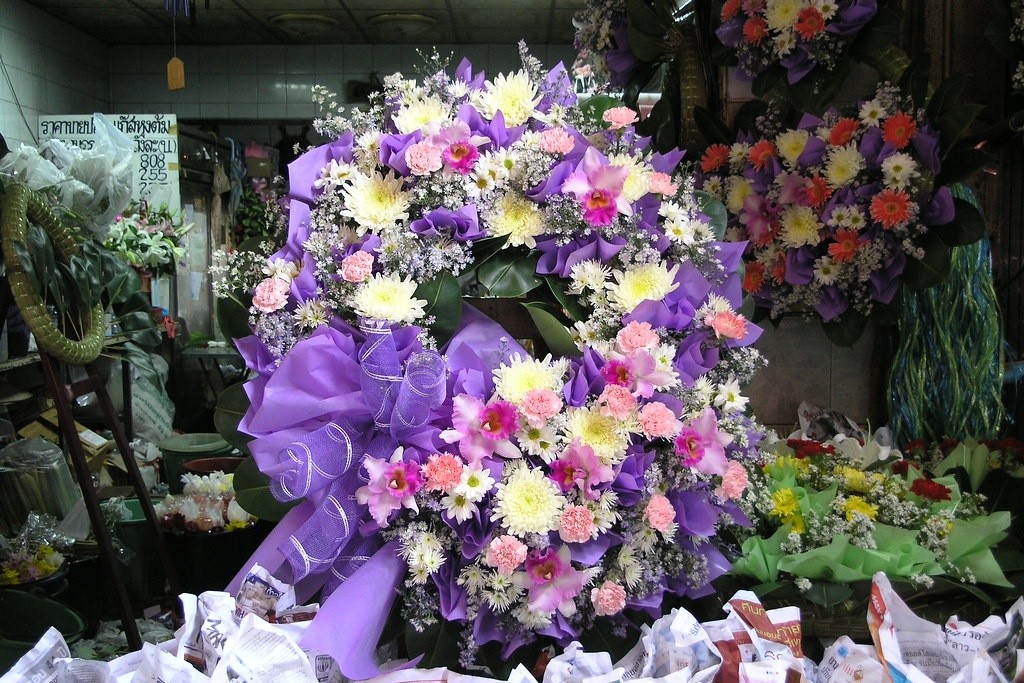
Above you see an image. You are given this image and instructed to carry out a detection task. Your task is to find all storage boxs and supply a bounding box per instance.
[16,405,112,483]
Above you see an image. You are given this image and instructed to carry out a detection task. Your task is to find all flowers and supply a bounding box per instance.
[0,0,1024,683]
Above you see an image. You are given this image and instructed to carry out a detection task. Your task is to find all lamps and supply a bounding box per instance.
[270,13,341,44]
[367,12,437,43]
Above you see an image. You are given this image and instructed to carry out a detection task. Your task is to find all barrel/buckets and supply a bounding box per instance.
[0,589,89,678]
[107,499,159,559]
[159,432,246,495]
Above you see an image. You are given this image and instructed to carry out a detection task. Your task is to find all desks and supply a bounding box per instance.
[180,347,251,403]
